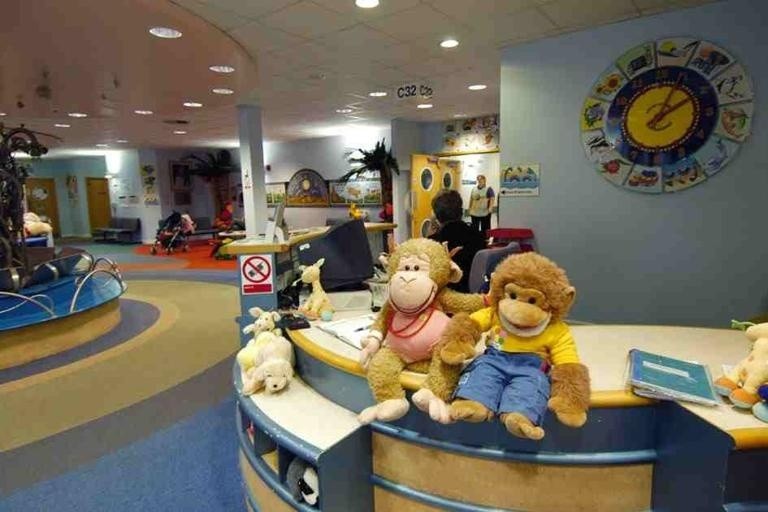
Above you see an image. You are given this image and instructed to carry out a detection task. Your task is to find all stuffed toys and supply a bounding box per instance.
[297,256,335,321]
[716,323,768,408]
[242,333,296,395]
[211,200,234,232]
[356,238,487,427]
[21,211,53,240]
[243,305,281,335]
[284,456,319,505]
[440,251,590,439]
[750,387,768,423]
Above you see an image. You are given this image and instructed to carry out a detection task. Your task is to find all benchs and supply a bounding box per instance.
[158,214,221,252]
[95,215,142,242]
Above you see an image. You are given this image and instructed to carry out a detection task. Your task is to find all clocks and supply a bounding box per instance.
[581,37,757,197]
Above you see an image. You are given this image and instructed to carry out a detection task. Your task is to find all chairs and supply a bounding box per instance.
[467,241,522,297]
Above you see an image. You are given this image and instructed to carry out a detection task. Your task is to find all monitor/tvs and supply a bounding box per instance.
[295,216,375,293]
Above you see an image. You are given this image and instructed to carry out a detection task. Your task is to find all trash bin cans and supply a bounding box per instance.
[17,236,48,247]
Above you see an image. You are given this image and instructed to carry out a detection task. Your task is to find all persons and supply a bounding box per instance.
[468,173,495,230]
[424,187,486,293]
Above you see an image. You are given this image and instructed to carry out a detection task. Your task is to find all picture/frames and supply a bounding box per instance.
[169,159,195,191]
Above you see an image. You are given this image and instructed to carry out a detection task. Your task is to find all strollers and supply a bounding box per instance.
[149,209,197,256]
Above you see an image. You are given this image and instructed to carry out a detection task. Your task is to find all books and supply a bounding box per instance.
[628,348,720,407]
[630,385,674,402]
[314,313,376,348]
